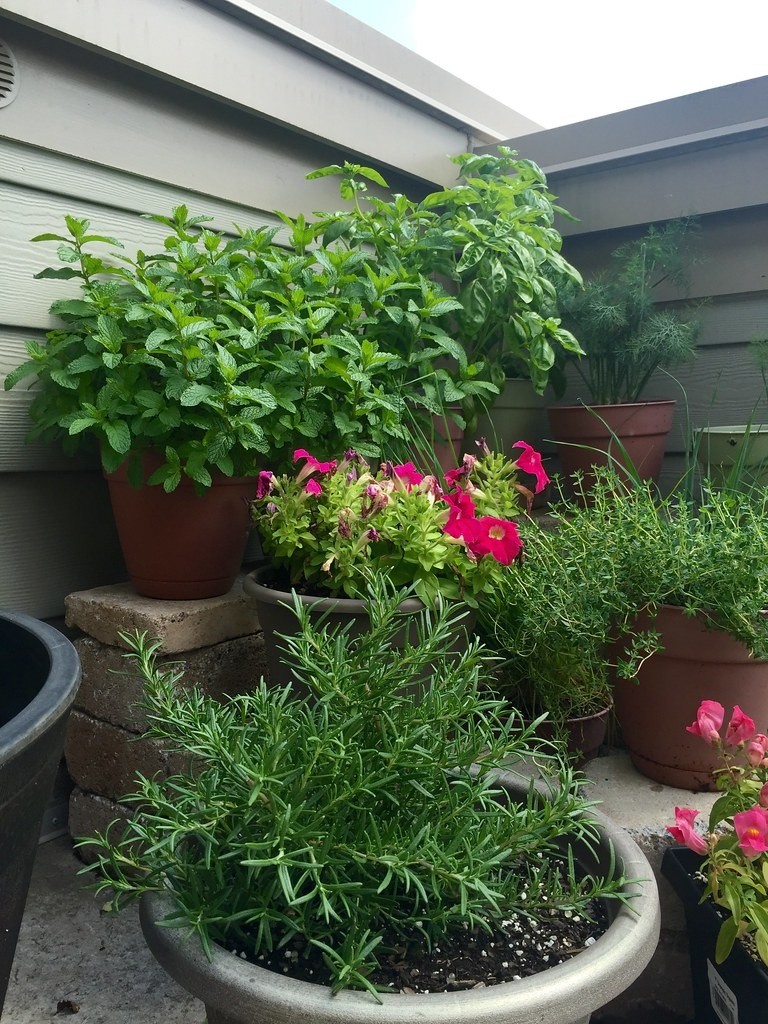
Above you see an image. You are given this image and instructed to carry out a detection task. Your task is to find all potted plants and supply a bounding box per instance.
[4,146,712,600]
[478,367,768,793]
[71,565,662,1024]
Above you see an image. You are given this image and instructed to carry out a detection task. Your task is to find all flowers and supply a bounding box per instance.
[665,700,768,969]
[242,437,551,611]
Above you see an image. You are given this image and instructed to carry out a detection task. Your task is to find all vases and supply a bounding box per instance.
[0,611,81,1019]
[661,845,768,1024]
[243,564,477,710]
[694,424,768,523]
